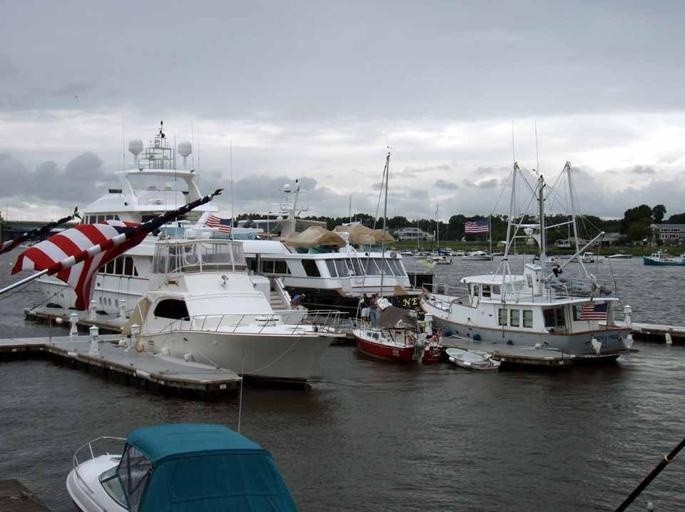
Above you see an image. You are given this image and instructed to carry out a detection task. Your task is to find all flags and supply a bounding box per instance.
[204,215,232,233]
[580,304,607,320]
[11,194,213,308]
[464,217,488,233]
[0,213,73,254]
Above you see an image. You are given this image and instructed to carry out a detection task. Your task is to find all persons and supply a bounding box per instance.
[409,325,442,346]
[369,291,380,320]
[291,293,306,310]
[360,298,369,321]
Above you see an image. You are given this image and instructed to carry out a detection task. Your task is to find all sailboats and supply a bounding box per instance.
[352,149,443,366]
[526,122,616,300]
[420,119,632,372]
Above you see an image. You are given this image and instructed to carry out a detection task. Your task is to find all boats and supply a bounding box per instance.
[33,118,219,321]
[446,348,501,372]
[135,213,346,382]
[230,178,428,313]
[394,244,685,267]
[65,424,297,511]
[390,227,432,240]
